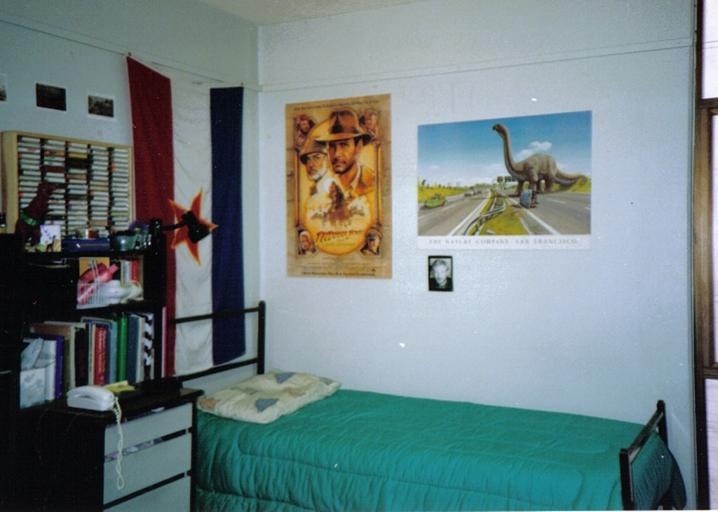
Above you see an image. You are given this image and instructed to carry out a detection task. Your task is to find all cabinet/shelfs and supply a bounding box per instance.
[0,237,166,406]
[0,131,135,238]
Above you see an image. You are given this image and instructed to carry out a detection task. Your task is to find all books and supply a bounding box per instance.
[17,134,134,249]
[11,307,167,403]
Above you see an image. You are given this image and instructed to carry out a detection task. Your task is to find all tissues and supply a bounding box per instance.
[19,337,46,409]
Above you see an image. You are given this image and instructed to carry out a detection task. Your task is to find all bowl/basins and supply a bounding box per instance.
[62,238,111,253]
[108,231,138,252]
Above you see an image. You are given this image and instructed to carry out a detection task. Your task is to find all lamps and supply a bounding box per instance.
[148,210,210,251]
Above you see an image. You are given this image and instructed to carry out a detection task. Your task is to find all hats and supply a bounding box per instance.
[367,229,382,241]
[299,136,329,164]
[314,107,371,146]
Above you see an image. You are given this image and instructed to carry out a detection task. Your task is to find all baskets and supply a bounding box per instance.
[78,281,111,310]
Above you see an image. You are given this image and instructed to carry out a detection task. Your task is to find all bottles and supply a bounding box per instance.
[14,181,55,235]
[77,262,118,304]
[134,225,149,250]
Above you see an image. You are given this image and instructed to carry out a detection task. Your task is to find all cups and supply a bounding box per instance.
[74,229,99,240]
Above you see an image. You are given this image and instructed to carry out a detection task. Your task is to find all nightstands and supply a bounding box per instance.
[0,386,204,512]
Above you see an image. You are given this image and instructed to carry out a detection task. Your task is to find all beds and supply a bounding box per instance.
[167,297,689,512]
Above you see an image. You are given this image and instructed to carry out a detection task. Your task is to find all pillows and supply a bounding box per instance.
[193,369,342,423]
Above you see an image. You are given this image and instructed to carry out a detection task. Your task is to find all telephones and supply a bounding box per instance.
[66,385,116,413]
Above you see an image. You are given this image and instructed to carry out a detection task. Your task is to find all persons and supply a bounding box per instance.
[519,187,532,207]
[294,108,383,257]
[429,259,451,289]
